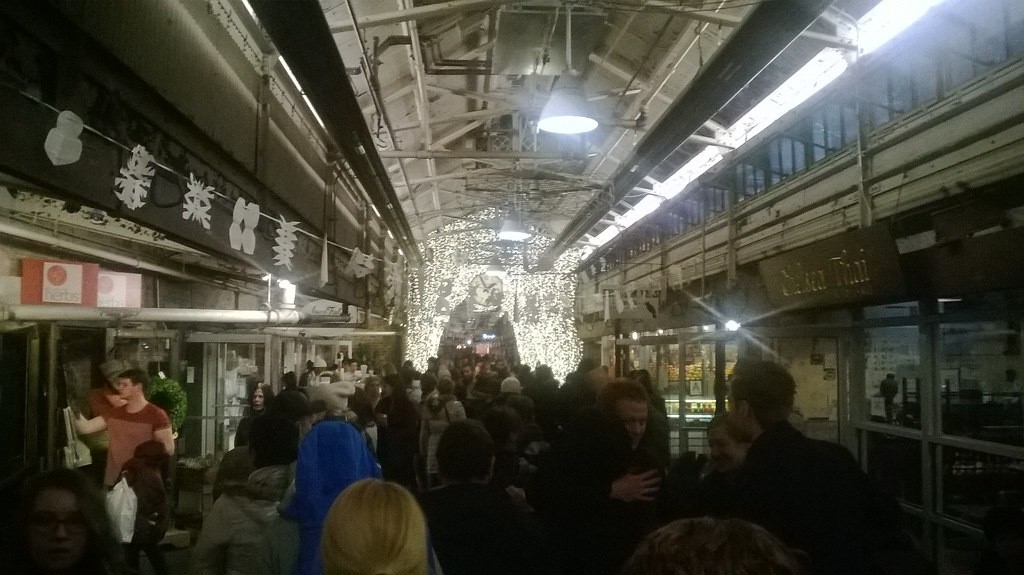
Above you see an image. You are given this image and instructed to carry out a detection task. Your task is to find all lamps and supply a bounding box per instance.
[499,209,531,240]
[538,3,598,134]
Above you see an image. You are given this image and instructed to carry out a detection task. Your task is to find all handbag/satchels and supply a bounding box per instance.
[62,407,92,468]
[103,476,137,544]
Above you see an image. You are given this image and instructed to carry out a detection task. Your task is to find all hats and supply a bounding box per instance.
[499,377,523,393]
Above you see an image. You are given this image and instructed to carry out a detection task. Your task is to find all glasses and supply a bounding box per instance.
[27,517,86,535]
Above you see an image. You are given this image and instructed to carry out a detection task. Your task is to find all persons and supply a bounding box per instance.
[974,506,1024,575]
[188,346,907,575]
[0,469,137,575]
[320,479,429,575]
[623,521,800,575]
[68,368,175,575]
